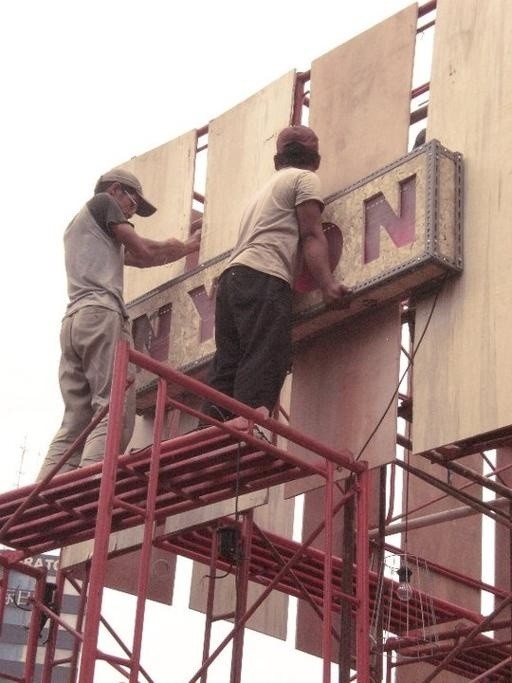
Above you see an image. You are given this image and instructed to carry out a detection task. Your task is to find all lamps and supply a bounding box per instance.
[396,565,413,602]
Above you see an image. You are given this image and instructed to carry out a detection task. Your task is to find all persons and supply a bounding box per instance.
[198,127,352,430]
[34,169,201,483]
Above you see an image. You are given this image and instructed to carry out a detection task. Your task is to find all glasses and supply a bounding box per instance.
[121,185,138,209]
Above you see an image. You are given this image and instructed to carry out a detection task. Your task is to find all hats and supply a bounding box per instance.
[98,169,156,216]
[277,126,318,155]
